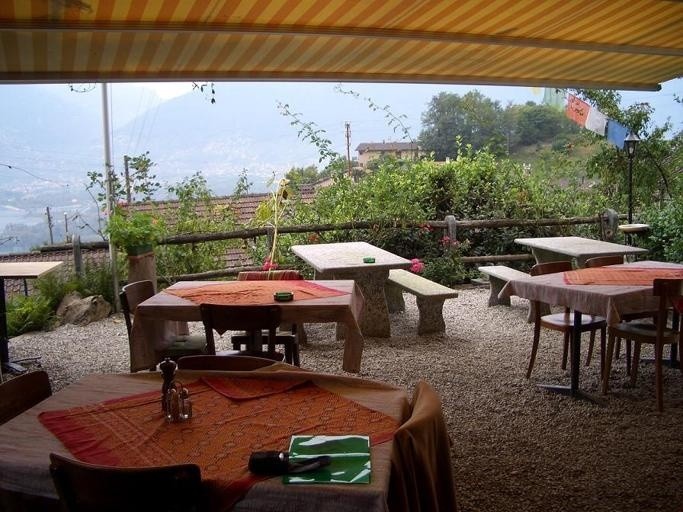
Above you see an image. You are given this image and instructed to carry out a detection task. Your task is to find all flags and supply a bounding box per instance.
[565,93,590,129]
[585,108,607,137]
[528,87,541,96]
[542,88,566,114]
[607,119,628,150]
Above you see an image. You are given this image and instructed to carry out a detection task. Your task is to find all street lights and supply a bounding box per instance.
[623,125,642,246]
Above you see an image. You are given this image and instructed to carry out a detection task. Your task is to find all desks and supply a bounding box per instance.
[127,280,368,374]
[0,367,416,512]
[494,259,682,405]
[287,241,415,342]
[0,261,64,374]
[511,234,650,324]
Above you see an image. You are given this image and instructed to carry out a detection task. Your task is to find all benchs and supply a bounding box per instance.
[383,264,458,337]
[476,265,553,327]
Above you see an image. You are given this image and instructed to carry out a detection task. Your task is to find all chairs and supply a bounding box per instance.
[44,451,210,512]
[393,378,445,512]
[196,266,301,367]
[115,278,210,374]
[0,368,51,428]
[599,279,681,415]
[524,260,607,389]
[173,349,279,374]
